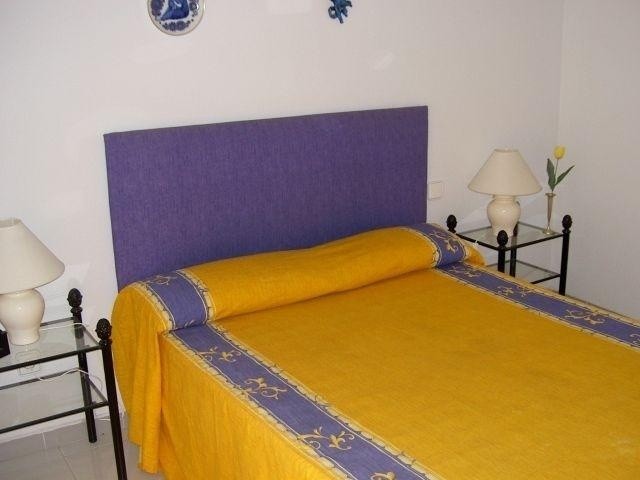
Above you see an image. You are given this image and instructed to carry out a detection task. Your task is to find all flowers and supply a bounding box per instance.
[546,146,575,194]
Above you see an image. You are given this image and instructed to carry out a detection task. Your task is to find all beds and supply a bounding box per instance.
[103,106,640,480]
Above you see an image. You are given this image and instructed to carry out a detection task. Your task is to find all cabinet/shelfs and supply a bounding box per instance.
[0,288,129,480]
[447,201,573,296]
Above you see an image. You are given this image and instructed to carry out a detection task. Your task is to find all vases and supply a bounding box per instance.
[543,193,556,235]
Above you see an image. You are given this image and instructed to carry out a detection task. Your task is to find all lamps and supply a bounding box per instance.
[467,148,543,238]
[0,218,66,346]
